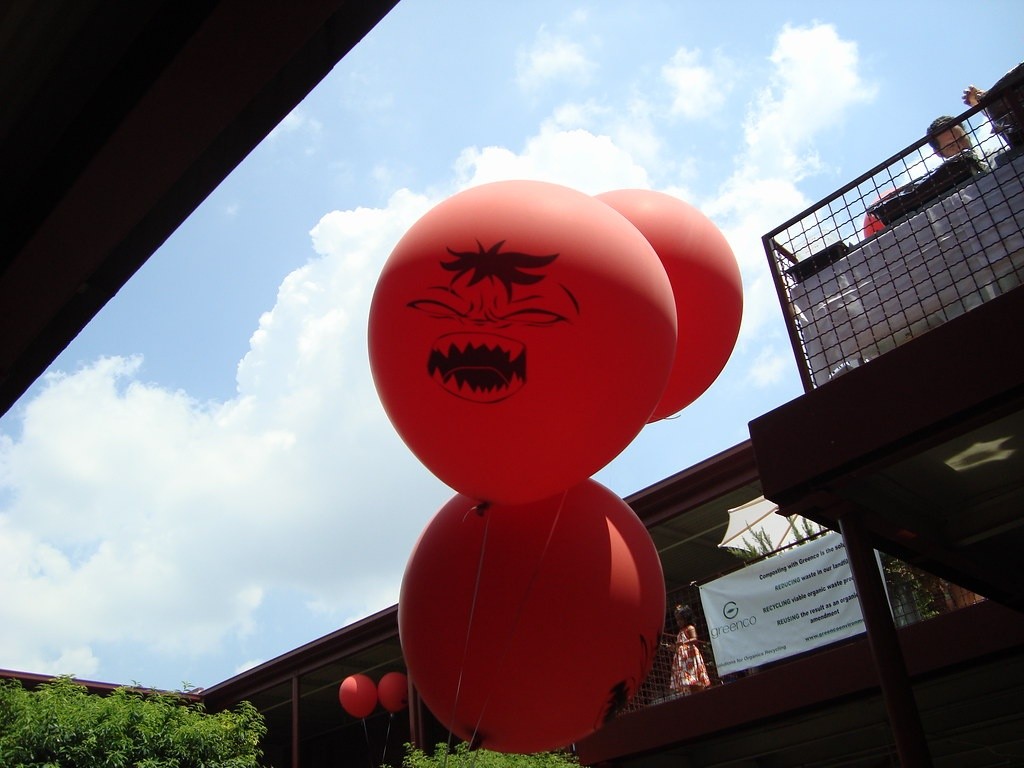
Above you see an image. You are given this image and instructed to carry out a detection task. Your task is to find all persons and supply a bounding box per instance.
[927,84,1010,172]
[670,602,712,696]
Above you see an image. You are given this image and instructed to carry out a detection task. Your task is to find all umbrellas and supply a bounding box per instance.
[717,496,835,558]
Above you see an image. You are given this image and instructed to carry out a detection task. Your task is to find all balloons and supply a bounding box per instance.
[863,190,899,239]
[341,183,743,754]
